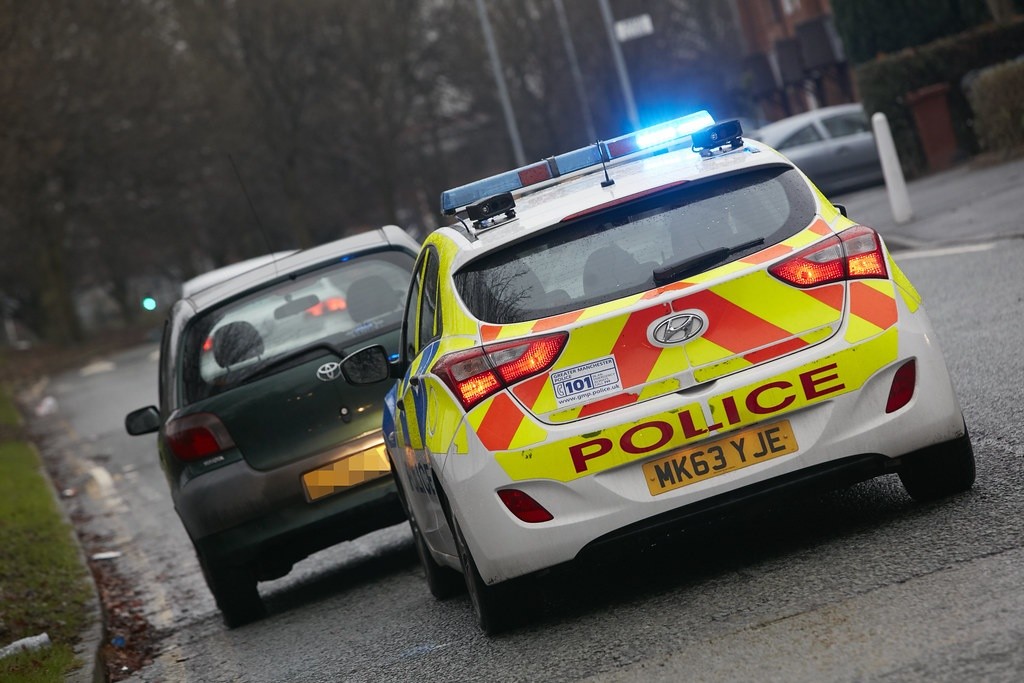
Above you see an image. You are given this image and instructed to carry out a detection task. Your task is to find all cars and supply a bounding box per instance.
[126,225,444,628]
[338,107,975,637]
[178,247,363,383]
[741,101,889,201]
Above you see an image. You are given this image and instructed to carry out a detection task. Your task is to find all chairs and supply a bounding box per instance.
[475,260,572,318]
[211,321,265,374]
[660,208,738,265]
[583,246,640,299]
[346,276,404,323]
